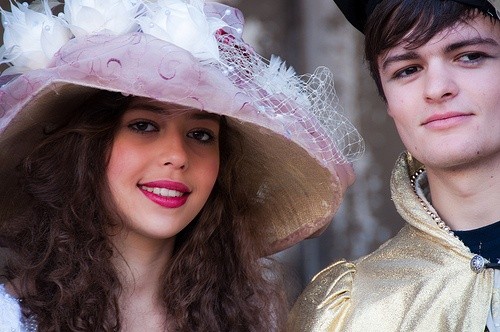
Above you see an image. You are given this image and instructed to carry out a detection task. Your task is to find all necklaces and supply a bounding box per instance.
[409,167,465,247]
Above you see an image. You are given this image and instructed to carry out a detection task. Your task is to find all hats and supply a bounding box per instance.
[334,0,500,32]
[0,0,367,257]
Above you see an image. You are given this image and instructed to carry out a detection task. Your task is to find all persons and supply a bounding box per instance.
[0,0,364,332]
[285,0,500,332]
